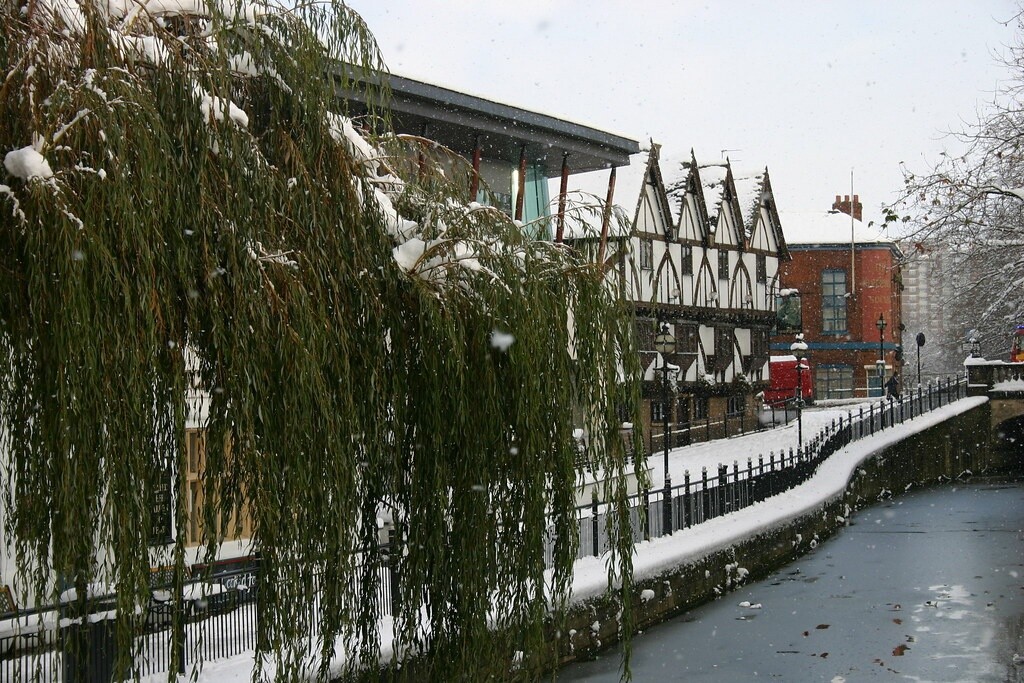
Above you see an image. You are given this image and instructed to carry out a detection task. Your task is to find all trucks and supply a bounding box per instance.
[766,355,815,410]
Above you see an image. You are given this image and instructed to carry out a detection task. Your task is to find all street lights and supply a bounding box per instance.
[876,312,887,396]
[652,319,679,485]
[790,332,809,449]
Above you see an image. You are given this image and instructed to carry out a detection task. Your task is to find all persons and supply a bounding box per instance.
[885,372,901,403]
